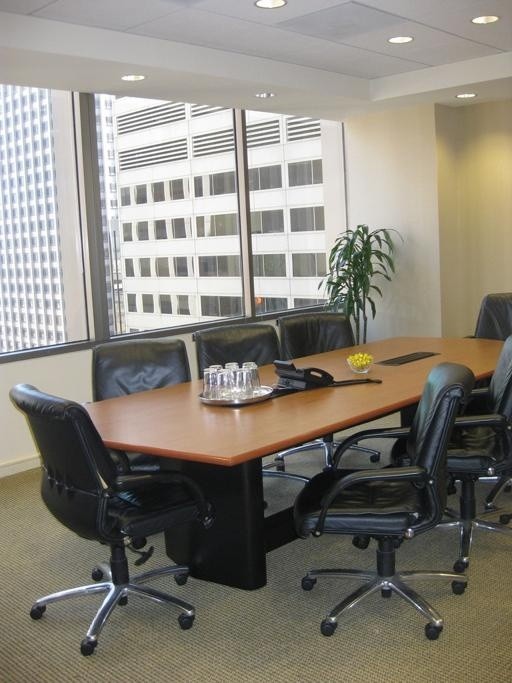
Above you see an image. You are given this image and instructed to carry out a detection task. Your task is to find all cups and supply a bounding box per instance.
[203,362,260,402]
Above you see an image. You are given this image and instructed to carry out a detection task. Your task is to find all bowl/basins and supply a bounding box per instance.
[347,359,374,374]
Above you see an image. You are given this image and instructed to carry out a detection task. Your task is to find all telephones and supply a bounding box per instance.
[273,360,334,391]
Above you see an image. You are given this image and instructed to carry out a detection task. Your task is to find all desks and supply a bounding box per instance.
[86,335,508,592]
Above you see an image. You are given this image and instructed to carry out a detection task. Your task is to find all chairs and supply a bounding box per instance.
[193,323,313,509]
[89,339,193,553]
[454,293,511,415]
[274,312,382,472]
[388,335,511,595]
[292,360,478,641]
[10,381,216,655]
